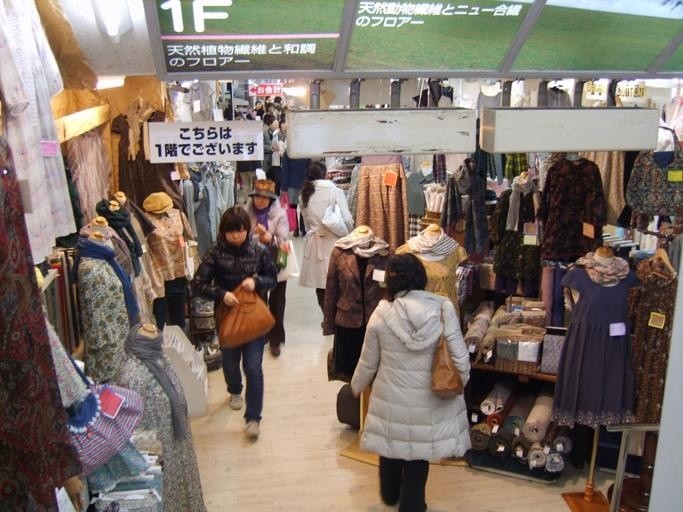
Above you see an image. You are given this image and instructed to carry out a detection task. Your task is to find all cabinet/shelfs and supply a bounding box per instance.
[160,326,208,416]
[462,355,579,484]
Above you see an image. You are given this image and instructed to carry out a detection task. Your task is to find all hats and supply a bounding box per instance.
[247,179,276,200]
[143,192,173,214]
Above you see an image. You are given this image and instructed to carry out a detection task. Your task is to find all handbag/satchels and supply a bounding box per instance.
[431,336,462,401]
[321,203,348,238]
[286,208,296,231]
[215,285,275,349]
[70,386,143,475]
[626,128,683,216]
[268,234,301,283]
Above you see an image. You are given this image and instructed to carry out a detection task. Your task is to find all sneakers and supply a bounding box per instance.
[229,393,242,410]
[244,420,258,440]
[270,346,279,356]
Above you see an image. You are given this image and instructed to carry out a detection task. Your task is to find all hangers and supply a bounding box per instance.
[647,234,675,280]
[599,223,642,250]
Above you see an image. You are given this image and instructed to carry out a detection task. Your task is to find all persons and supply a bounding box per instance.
[247,95,354,356]
[321,223,469,385]
[66,191,204,510]
[549,249,637,426]
[189,206,278,435]
[489,154,608,299]
[350,252,471,512]
